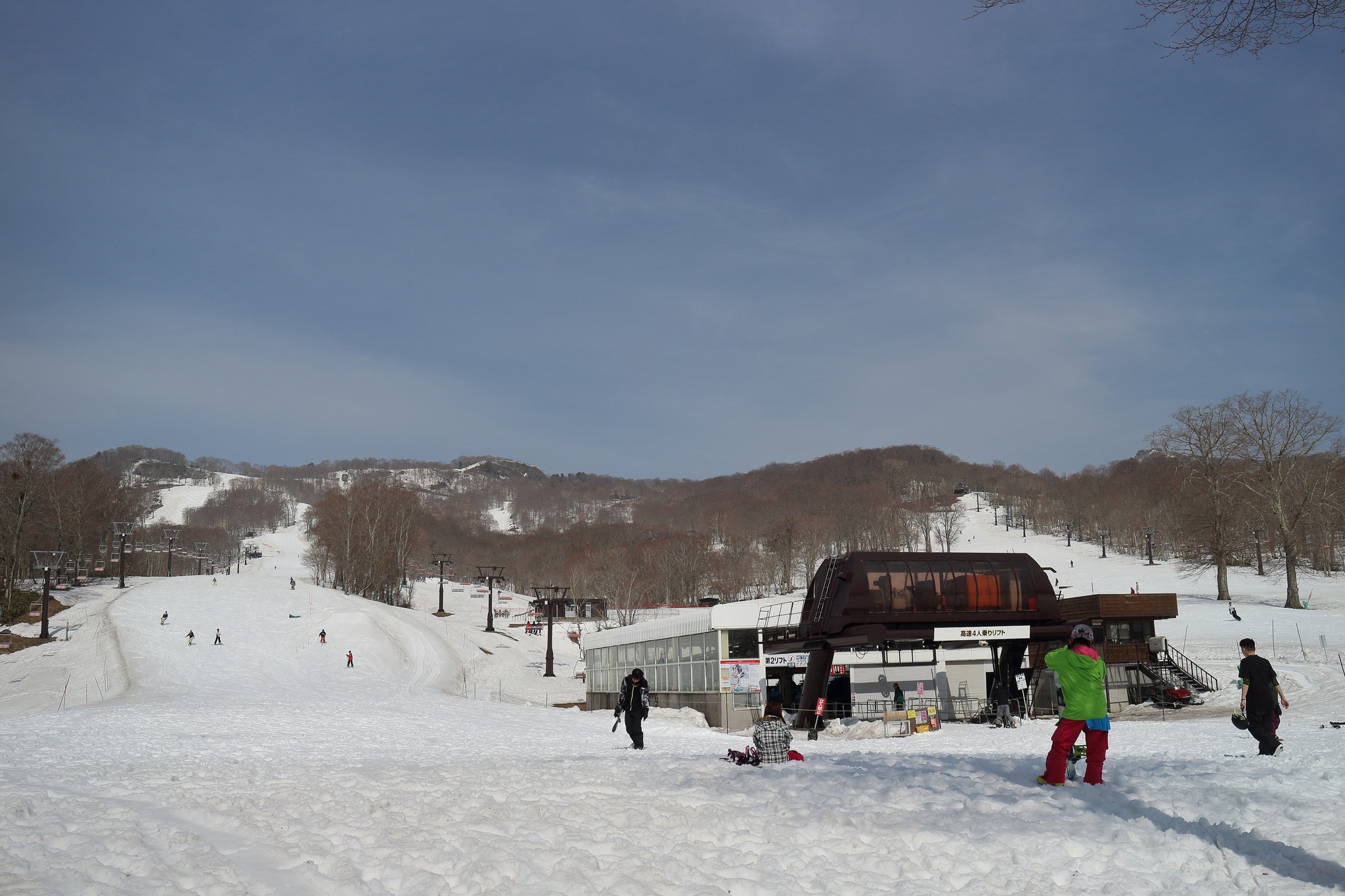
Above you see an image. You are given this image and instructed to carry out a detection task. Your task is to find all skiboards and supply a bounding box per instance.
[211,643,225,646]
[320,642,327,645]
[988,725,1018,729]
[159,624,165,625]
[290,589,295,590]
[345,666,354,670]
[212,585,217,586]
[186,643,196,646]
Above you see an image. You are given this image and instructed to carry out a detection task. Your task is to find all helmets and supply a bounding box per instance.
[1231,713,1250,729]
[996,682,1002,688]
[1071,624,1093,641]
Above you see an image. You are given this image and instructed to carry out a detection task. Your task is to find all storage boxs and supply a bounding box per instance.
[883,710,907,721]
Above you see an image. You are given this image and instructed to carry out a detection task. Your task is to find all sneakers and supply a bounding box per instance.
[1036,775,1064,787]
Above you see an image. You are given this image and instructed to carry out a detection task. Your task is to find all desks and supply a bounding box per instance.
[883,720,911,738]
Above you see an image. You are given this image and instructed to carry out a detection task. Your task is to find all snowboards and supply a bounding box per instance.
[1053,585,1058,587]
[611,697,626,733]
[1053,668,1078,782]
[1069,566,1075,568]
[522,631,544,639]
[580,682,586,684]
[1237,619,1243,622]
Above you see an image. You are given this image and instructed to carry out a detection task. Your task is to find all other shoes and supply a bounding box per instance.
[635,746,642,751]
[1271,743,1283,756]
[1012,722,1015,727]
[996,724,1000,728]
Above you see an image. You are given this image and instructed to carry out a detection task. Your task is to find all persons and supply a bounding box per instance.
[1036,624,1110,786]
[289,614,301,618]
[161,611,168,625]
[206,568,209,575]
[989,682,1017,728]
[346,650,353,668]
[1130,582,1139,594]
[582,668,586,683]
[409,575,426,582]
[289,577,296,589]
[214,629,221,645]
[1055,578,1058,586]
[1229,602,1241,621]
[319,629,326,644]
[753,689,793,763]
[525,621,542,634]
[1070,560,1073,567]
[494,609,508,618]
[213,577,217,585]
[1239,638,1289,756]
[614,668,650,749]
[185,630,195,645]
[960,531,975,543]
[893,683,903,711]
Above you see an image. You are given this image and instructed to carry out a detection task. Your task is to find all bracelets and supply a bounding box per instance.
[1241,699,1245,701]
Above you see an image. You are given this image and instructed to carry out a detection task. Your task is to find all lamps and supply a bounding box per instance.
[978,640,988,646]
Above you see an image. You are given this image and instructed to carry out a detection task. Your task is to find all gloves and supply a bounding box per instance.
[643,708,649,721]
[619,698,624,707]
